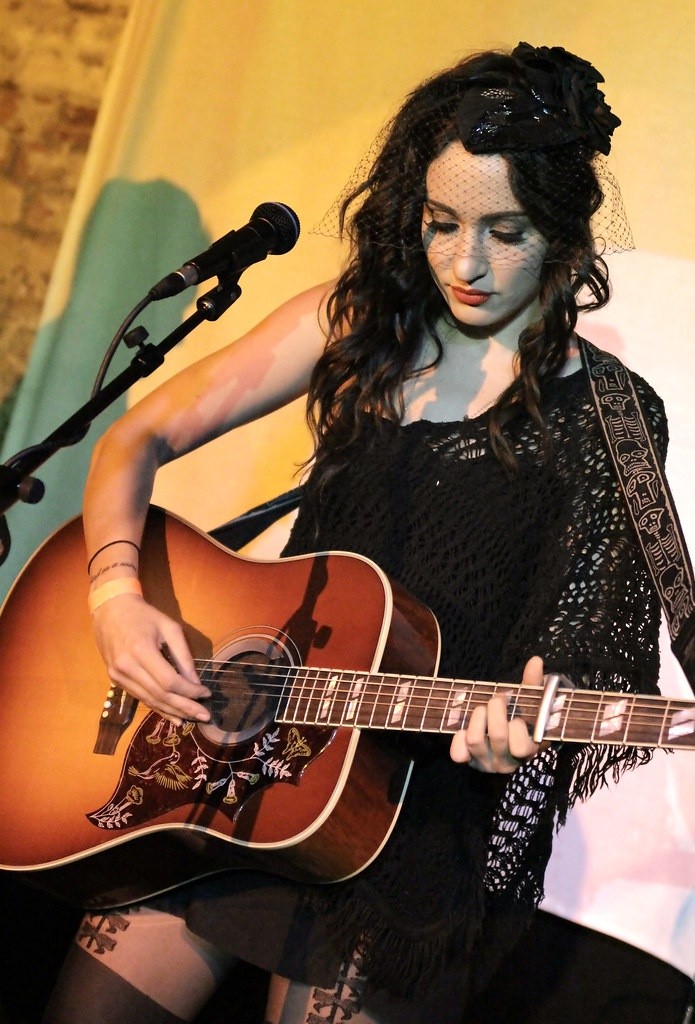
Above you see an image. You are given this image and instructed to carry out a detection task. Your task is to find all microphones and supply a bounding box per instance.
[149,202,301,302]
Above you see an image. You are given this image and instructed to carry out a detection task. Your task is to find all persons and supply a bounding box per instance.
[35,39,669,1017]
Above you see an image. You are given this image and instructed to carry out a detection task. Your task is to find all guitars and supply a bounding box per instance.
[0,502,695,912]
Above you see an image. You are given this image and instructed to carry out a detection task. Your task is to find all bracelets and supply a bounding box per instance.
[87,539,140,574]
[88,577,144,615]
